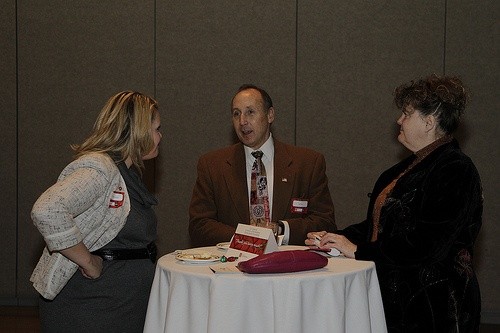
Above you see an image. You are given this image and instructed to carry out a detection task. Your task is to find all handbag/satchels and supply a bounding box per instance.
[237,248,332,274]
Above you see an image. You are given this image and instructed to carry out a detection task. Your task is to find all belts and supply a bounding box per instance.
[91,242,158,264]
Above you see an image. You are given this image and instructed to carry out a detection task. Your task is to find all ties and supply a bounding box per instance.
[250,151,272,224]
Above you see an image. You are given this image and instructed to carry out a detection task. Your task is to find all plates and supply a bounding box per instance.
[217,241,229,249]
[177,253,220,261]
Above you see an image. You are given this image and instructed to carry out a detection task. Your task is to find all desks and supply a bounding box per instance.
[142,244,389,333]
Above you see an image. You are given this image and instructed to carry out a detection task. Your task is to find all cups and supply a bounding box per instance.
[256,222,279,241]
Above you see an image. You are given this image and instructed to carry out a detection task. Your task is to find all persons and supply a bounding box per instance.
[305,74,483,333]
[188,84,336,246]
[30,88,162,333]
